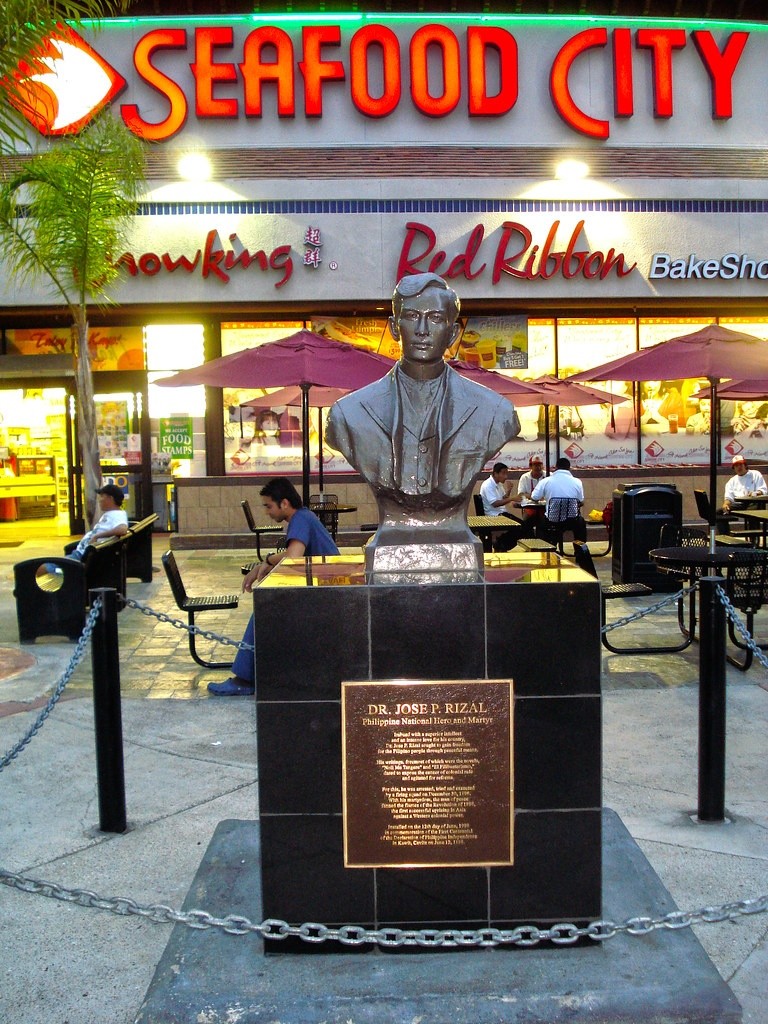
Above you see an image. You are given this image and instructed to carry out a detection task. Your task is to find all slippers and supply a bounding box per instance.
[208,678,254,695]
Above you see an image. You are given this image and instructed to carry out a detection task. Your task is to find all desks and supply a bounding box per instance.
[730,510,768,530]
[734,496,768,509]
[648,546,768,577]
[311,505,358,527]
[467,516,520,553]
[513,501,546,538]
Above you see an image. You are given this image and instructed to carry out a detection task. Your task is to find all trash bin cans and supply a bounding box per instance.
[613,481,683,592]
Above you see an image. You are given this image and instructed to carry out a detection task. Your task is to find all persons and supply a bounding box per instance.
[322,273,520,513]
[480,462,533,552]
[206,476,341,697]
[35,484,128,576]
[530,457,589,546]
[716,455,767,536]
[250,411,283,462]
[517,454,554,536]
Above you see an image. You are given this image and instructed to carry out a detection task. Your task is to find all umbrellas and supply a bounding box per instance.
[692,380,768,404]
[502,374,632,480]
[149,328,399,508]
[564,321,768,532]
[445,356,559,396]
[239,385,371,491]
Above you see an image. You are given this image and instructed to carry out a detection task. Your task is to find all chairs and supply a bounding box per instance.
[161,489,768,671]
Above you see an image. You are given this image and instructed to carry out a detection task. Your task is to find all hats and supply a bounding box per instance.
[94,484,124,505]
[530,455,542,464]
[732,455,746,466]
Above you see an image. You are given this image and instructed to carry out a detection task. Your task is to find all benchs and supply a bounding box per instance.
[705,534,752,547]
[517,539,557,552]
[13,510,159,644]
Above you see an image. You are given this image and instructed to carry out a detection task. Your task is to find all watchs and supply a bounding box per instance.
[265,552,276,566]
